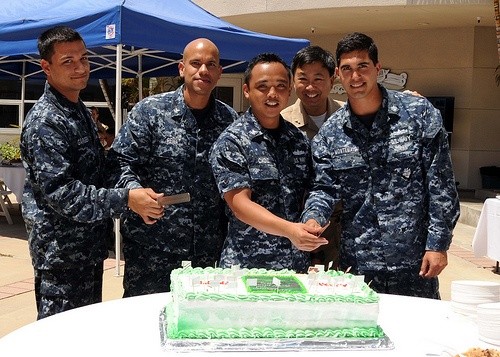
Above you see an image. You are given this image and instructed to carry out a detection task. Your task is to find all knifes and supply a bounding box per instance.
[314,220,331,237]
[157,193,191,206]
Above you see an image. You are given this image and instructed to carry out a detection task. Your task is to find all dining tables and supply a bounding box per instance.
[0,293,500,357]
[0,163,26,204]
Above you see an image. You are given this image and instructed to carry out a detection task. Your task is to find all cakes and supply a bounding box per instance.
[164,265,384,339]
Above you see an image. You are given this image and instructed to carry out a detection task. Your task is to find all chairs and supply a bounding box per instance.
[0,181,15,225]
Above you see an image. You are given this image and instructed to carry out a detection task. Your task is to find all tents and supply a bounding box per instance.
[0,0,314,159]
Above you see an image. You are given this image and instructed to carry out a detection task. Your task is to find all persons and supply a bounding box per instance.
[106,37,241,304]
[301,30,462,299]
[18,26,165,322]
[208,53,329,274]
[280,46,348,140]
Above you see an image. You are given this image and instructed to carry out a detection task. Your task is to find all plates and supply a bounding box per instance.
[459,349,500,357]
[451,281,500,347]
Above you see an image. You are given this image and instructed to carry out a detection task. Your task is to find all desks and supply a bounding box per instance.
[472,198,500,272]
